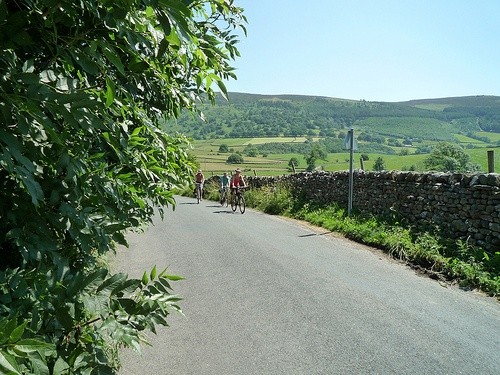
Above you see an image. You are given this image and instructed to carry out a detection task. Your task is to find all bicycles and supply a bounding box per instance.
[219,186,230,207]
[231,187,246,214]
[194,182,202,204]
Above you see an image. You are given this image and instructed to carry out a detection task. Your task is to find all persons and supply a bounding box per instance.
[229,169,247,207]
[194,170,204,201]
[219,172,231,204]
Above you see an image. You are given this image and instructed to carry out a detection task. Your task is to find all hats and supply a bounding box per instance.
[235,168,241,173]
[198,170,202,174]
